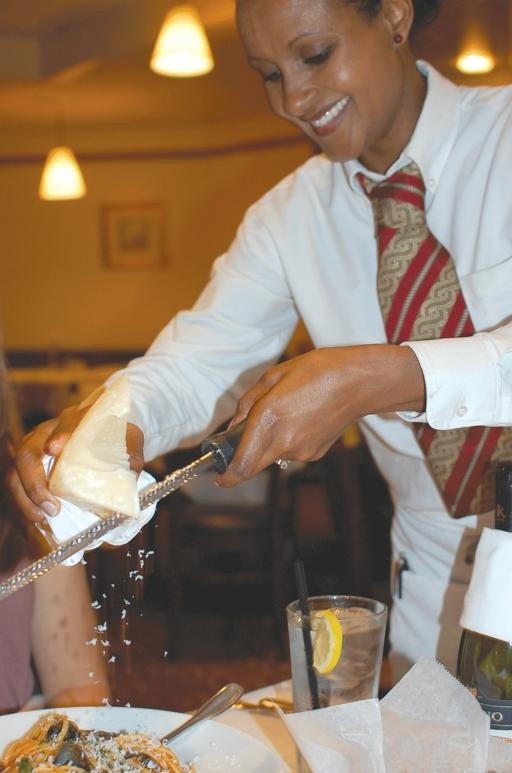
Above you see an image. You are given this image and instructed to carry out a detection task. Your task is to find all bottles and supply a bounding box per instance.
[451,462,511,737]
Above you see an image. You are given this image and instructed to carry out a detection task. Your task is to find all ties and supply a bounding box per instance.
[354,160,512,522]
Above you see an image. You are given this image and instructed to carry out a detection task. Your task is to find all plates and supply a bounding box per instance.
[0,707,290,773]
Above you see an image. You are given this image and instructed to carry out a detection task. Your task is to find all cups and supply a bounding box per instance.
[286,596,388,709]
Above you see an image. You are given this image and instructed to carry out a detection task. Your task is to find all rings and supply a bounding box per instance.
[276,457,290,470]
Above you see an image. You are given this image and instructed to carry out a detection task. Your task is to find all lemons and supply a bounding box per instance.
[312,609,343,674]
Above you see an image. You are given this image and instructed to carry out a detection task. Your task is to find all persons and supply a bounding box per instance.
[1,427,111,710]
[1,0,510,691]
